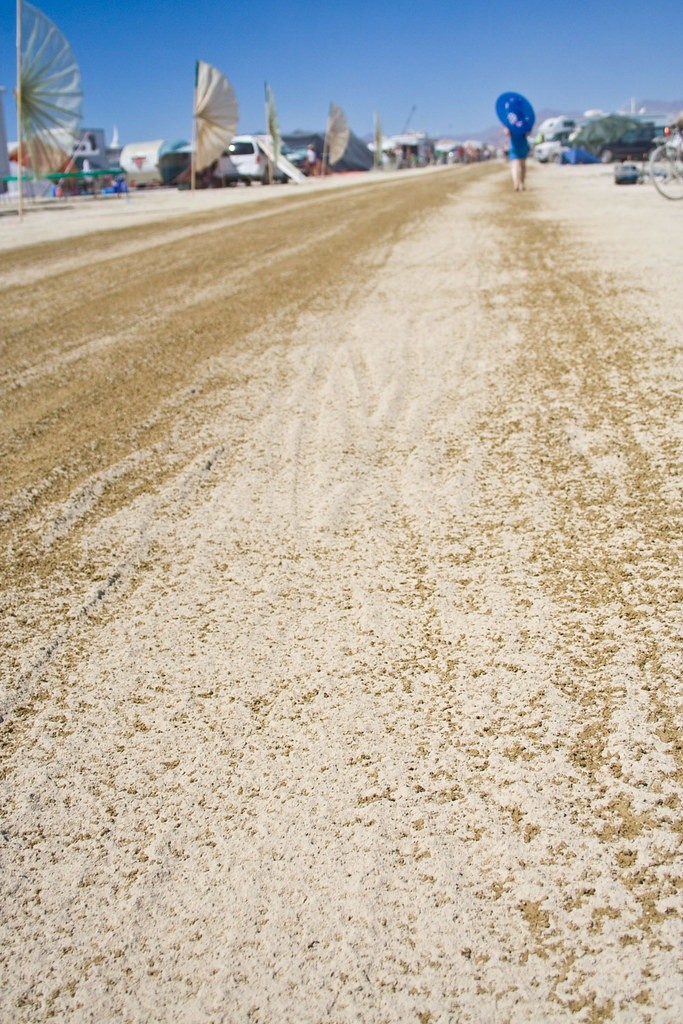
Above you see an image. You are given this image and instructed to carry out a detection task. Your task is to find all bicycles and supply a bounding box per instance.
[646,120,683,201]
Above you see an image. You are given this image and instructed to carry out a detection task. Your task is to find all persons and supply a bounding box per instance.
[307,144,317,178]
[494,128,534,192]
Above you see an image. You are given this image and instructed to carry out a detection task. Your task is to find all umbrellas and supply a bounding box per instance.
[494,92,536,133]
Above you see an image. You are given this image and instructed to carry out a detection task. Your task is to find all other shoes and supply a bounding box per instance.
[514,184,524,192]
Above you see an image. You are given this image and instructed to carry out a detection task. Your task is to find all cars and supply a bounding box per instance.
[212,135,292,186]
[614,163,640,185]
[281,129,332,173]
